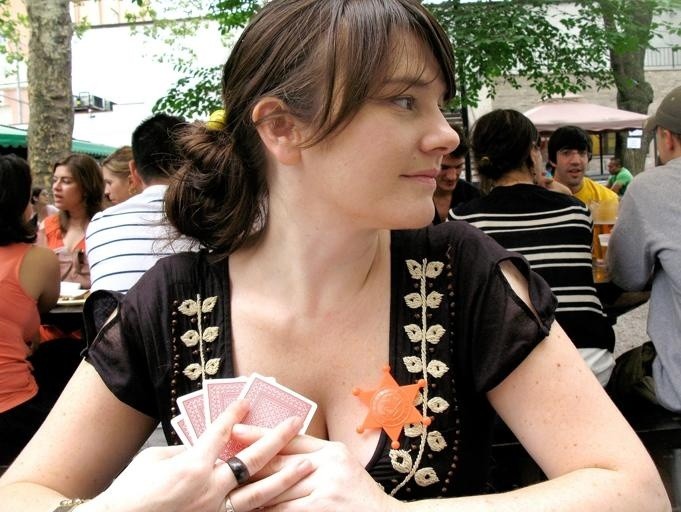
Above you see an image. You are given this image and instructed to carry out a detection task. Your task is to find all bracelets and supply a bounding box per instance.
[52,490,90,512]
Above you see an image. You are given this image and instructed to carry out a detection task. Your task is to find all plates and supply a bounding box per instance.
[55,288,90,307]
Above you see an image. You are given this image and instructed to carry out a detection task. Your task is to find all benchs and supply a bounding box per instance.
[487,396,681,491]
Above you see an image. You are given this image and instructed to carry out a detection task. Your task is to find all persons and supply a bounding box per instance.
[446,110,617,389]
[602,87,681,462]
[0,0,681,512]
[544,124,621,283]
[605,156,635,195]
[1,112,206,470]
[432,123,488,226]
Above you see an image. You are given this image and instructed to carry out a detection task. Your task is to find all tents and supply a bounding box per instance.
[524,100,650,175]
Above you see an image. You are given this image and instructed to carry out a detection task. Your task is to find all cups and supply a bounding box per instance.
[591,222,616,267]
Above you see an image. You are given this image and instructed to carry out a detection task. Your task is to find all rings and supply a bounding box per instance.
[227,453,253,488]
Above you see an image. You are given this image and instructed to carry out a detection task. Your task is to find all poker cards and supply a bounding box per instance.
[171,372,318,466]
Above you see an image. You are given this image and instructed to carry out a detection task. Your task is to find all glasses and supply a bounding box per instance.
[556,149,591,161]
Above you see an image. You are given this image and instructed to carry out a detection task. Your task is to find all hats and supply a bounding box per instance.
[648,85,681,134]
